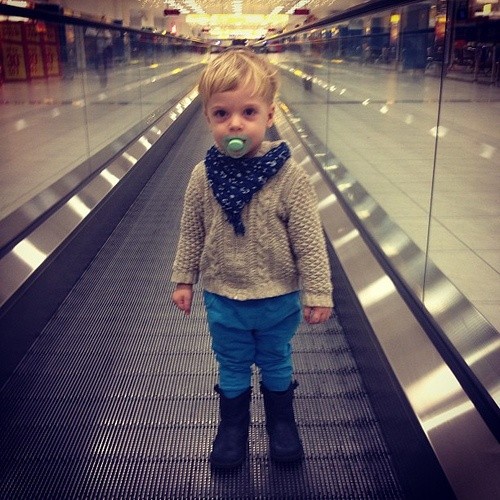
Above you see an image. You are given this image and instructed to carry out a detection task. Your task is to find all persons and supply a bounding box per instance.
[301,42,316,93]
[92,38,108,89]
[169,45,336,478]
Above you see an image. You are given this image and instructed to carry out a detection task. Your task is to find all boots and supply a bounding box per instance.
[209,384,253,472]
[258,380,305,464]
